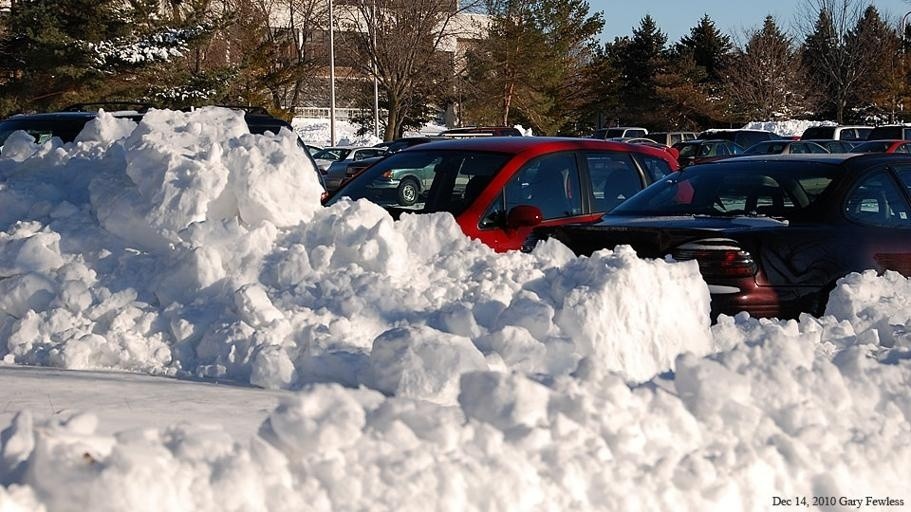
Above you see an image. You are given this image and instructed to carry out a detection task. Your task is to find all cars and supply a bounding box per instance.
[0,101,328,205]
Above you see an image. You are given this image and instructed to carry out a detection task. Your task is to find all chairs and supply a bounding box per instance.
[603,168,639,207]
[446,174,506,215]
[849,190,891,225]
[746,188,781,220]
